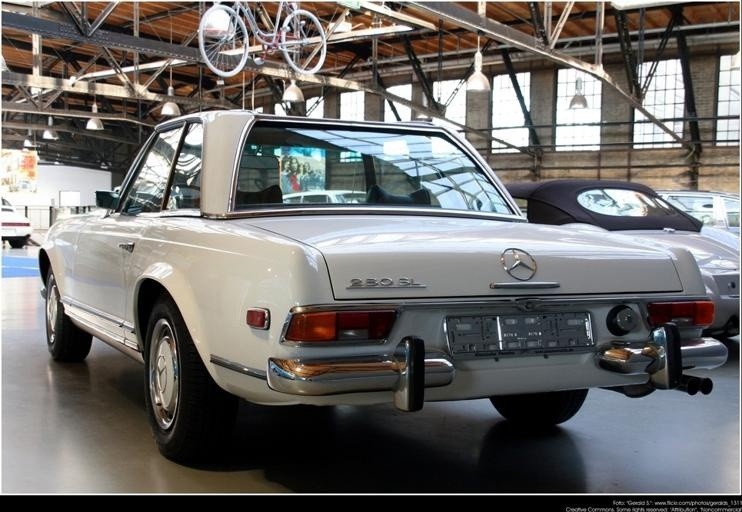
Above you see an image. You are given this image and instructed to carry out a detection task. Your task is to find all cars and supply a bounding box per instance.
[1,196,32,250]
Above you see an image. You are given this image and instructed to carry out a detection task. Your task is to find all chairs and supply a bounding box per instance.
[366,185,431,206]
[236,184,283,205]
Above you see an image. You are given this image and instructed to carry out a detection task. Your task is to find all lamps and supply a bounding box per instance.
[25,2,184,150]
[280,78,306,103]
[465,32,491,92]
[568,67,589,110]
[198,2,235,38]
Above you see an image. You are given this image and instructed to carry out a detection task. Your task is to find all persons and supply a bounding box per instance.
[280,155,325,193]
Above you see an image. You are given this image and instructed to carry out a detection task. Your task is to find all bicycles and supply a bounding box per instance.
[197,2,328,78]
